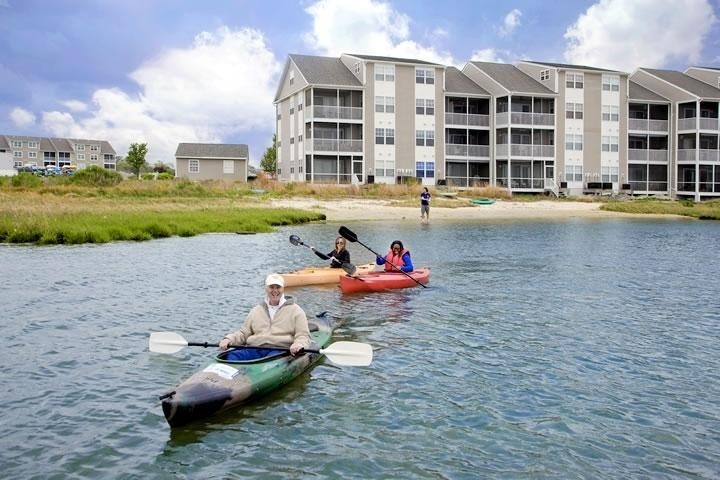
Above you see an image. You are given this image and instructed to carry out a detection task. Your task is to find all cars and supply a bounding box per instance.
[15,163,77,176]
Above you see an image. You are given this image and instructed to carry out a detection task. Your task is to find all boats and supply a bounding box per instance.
[469,197,494,205]
[337,265,430,293]
[275,261,376,287]
[158,311,334,428]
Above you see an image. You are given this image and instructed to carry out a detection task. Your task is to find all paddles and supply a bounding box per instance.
[290,235,356,274]
[149,332,373,367]
[339,227,427,288]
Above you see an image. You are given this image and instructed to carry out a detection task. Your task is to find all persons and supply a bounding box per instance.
[420,187,432,224]
[217,272,313,361]
[311,236,351,269]
[376,240,414,274]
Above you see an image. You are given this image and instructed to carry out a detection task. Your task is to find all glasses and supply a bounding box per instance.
[337,241,343,245]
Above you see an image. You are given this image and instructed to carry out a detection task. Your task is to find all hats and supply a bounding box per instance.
[264,274,283,287]
